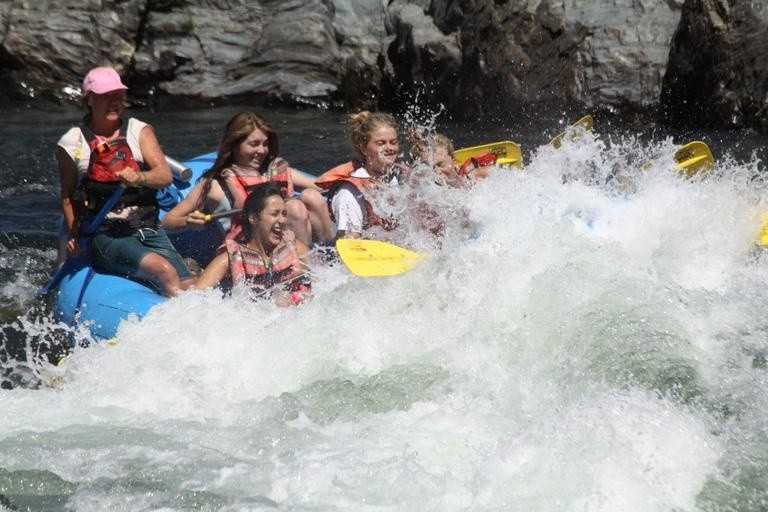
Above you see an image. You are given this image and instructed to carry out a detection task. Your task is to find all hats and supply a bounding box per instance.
[82,67,129,97]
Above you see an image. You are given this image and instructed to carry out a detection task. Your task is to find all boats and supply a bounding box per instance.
[55,150,329,349]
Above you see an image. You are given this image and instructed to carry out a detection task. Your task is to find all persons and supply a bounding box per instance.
[161,112,333,247]
[192,184,314,308]
[56,67,197,299]
[406,134,505,250]
[328,111,414,242]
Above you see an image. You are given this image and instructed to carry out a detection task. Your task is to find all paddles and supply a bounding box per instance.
[550,116,593,150]
[675,142,714,177]
[336,239,435,277]
[452,140,523,174]
[754,211,767,246]
